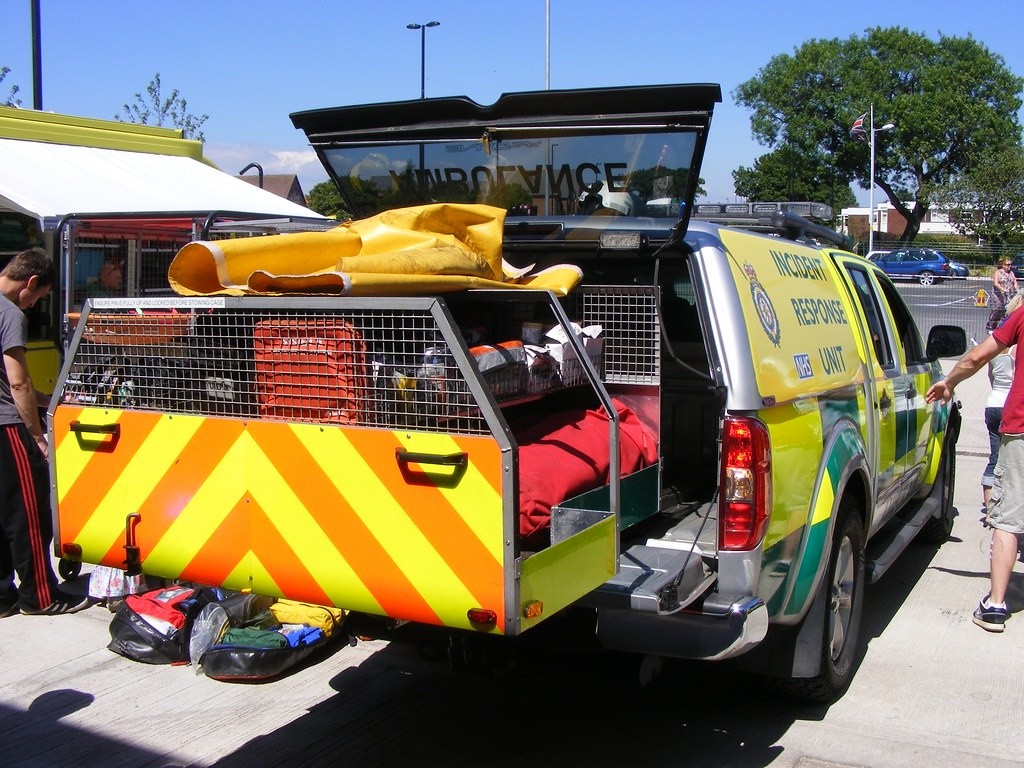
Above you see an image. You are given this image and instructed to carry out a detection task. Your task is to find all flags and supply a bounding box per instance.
[850,113,867,142]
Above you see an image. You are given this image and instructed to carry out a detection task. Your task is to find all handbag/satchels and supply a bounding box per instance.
[106,579,227,664]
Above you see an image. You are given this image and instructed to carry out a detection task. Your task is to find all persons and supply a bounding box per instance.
[0,248,86,616]
[926,255,1024,632]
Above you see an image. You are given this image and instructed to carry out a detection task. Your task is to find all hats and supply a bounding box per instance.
[98,264,115,281]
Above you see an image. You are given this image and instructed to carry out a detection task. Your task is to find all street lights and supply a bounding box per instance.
[407,21,440,190]
[867,123,895,255]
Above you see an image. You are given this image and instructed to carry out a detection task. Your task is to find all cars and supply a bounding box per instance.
[864,251,891,262]
[1011,256,1024,279]
[949,259,969,279]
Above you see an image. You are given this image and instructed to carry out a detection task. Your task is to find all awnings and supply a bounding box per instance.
[0,136,336,232]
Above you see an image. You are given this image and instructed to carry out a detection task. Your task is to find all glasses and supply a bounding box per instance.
[1004,264,1012,266]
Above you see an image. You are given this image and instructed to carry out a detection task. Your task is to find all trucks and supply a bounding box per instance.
[1,105,340,465]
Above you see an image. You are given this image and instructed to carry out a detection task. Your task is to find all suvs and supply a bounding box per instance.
[873,247,950,285]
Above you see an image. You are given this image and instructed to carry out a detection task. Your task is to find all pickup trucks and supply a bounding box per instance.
[288,80,965,700]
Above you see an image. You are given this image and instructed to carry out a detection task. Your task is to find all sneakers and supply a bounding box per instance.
[0,597,20,618]
[972,591,1007,632]
[20,589,88,614]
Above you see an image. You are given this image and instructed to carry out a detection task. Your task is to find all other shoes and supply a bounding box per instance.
[986,329,993,335]
